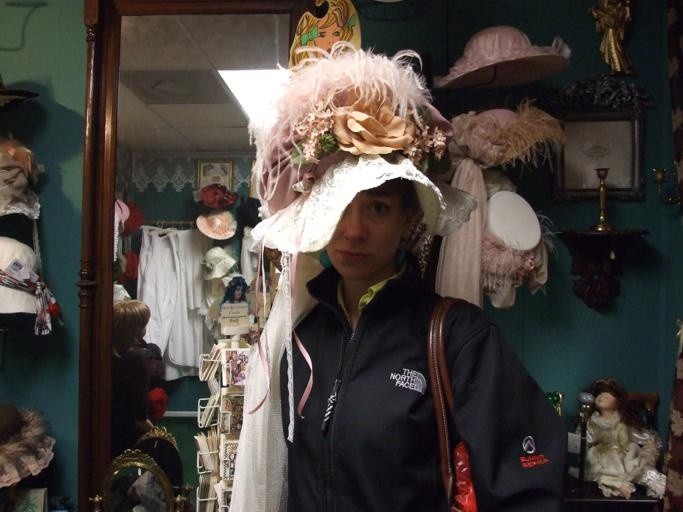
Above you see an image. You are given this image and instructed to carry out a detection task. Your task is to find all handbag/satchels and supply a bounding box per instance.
[441,440,479,512]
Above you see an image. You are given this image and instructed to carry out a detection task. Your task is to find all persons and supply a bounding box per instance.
[223,276,249,303]
[584,376,667,499]
[114,300,163,456]
[280,178,568,512]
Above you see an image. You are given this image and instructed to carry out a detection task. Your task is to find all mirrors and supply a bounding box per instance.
[90,449,187,512]
[76,0,324,512]
[132,426,194,498]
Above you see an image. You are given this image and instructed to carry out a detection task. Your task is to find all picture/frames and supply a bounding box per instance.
[550,108,647,203]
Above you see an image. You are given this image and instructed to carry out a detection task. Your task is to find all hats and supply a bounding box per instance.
[252,152,447,258]
[473,102,544,168]
[482,189,550,292]
[201,182,238,208]
[197,210,238,242]
[0,139,42,220]
[434,25,567,94]
[200,246,237,281]
[0,397,57,490]
[0,235,53,316]
